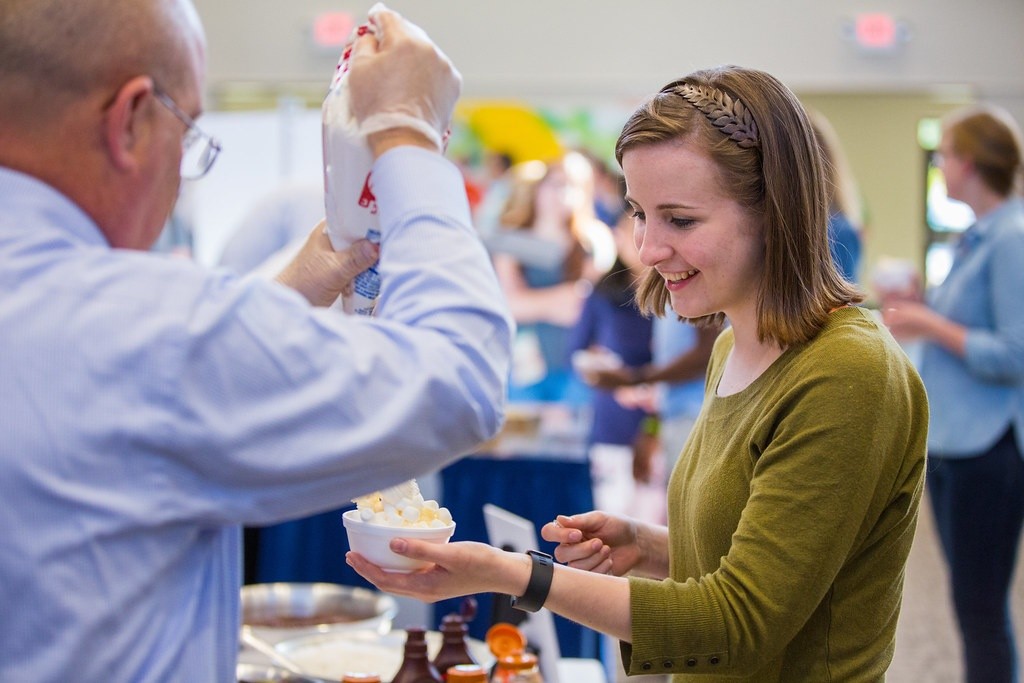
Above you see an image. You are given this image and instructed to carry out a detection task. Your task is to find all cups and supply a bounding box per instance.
[875,262,921,304]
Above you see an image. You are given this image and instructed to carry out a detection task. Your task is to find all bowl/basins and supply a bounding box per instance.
[342,509,457,577]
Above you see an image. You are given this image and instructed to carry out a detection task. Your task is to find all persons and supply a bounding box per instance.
[880,110,1024,683]
[345,61,928,683]
[1,1,517,683]
[250,103,868,667]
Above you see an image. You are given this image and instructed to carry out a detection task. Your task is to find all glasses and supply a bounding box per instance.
[100,77,221,181]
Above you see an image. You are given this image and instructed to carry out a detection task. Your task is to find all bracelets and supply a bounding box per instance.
[512,548,555,614]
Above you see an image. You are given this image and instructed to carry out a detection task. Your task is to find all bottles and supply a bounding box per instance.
[431,615,481,683]
[386,625,445,683]
